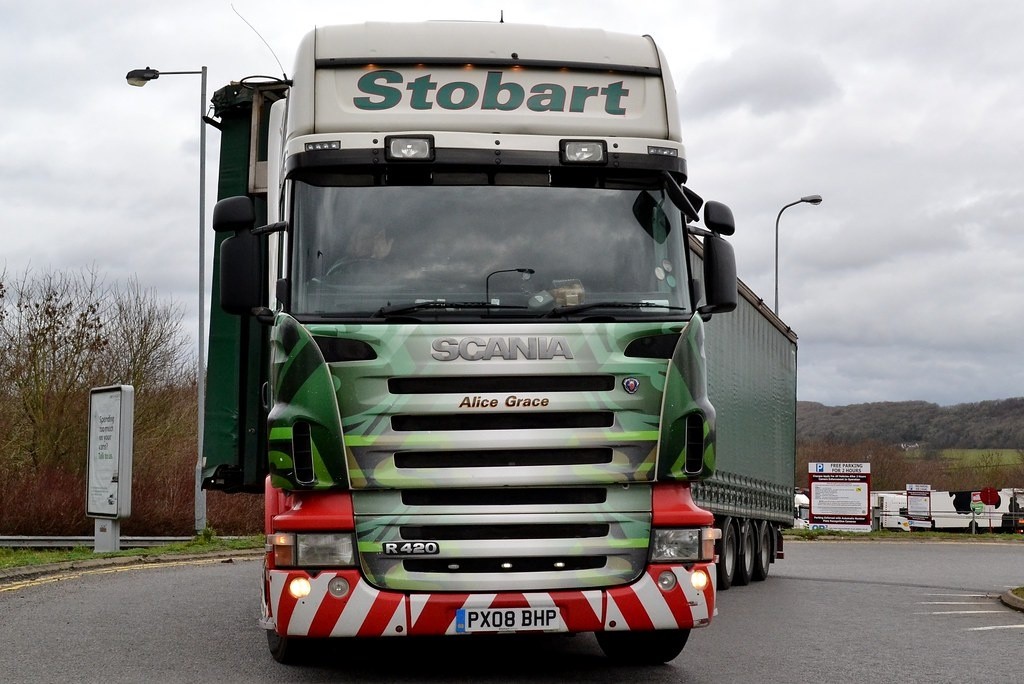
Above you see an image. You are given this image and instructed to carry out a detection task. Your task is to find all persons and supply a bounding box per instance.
[329,223,402,281]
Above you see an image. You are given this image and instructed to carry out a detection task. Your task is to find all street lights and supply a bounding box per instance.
[774,195,822,316]
[125,68,207,540]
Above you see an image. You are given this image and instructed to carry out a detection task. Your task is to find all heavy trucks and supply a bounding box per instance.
[792,494,809,529]
[882,488,1024,534]
[198,20,800,673]
